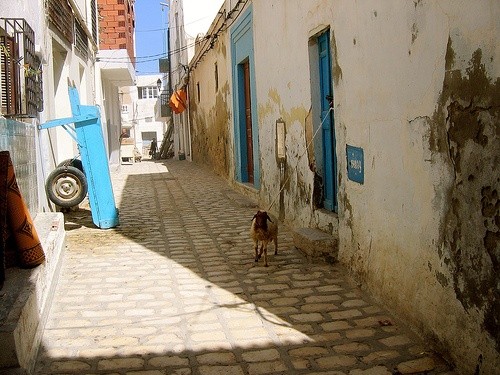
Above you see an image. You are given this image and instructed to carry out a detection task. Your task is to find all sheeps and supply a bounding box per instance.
[251,210,279,267]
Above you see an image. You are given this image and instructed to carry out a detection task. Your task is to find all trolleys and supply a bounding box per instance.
[37,76,120,229]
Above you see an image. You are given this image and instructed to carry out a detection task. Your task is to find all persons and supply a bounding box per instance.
[148,134,163,160]
[119,128,130,162]
